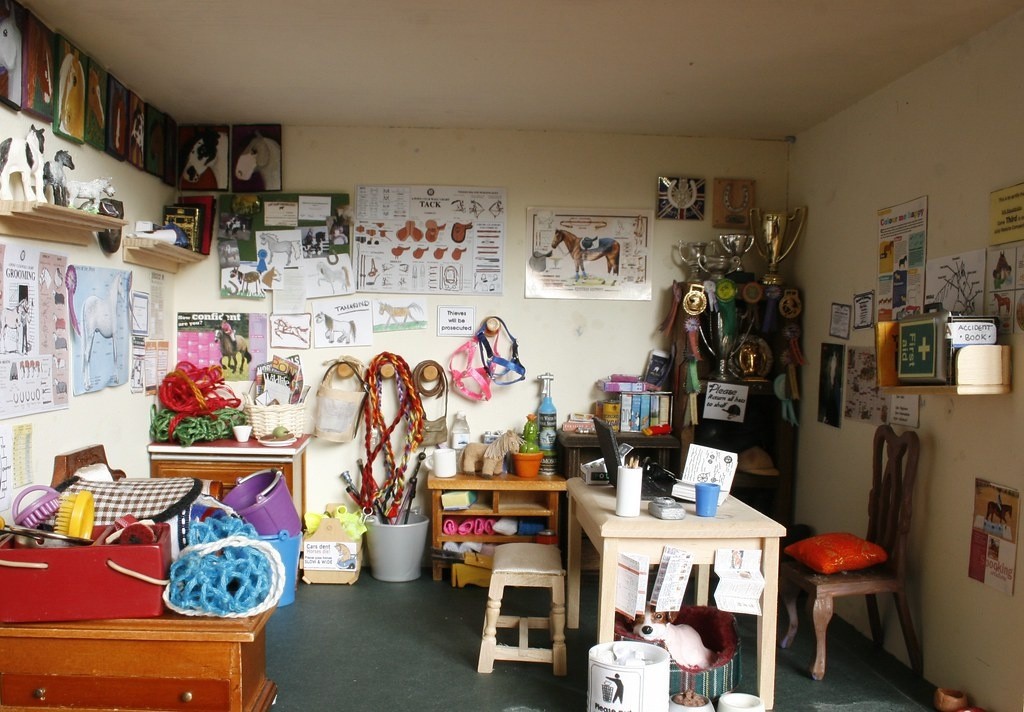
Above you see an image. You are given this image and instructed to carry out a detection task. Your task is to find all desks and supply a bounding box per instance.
[728,464,792,532]
[562,475,787,710]
[0,600,278,712]
[559,427,680,575]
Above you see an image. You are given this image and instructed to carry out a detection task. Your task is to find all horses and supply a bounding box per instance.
[459,428,526,479]
[552,229,620,286]
[43,150,75,207]
[66,177,116,210]
[0,124,48,202]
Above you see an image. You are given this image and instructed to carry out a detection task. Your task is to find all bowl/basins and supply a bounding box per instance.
[934,687,967,710]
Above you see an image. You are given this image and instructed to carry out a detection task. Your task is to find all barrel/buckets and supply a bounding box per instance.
[222,467,302,536]
[258,530,303,608]
[364,512,429,582]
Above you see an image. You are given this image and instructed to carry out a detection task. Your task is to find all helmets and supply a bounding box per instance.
[221,315,227,319]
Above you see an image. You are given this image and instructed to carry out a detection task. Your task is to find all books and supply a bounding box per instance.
[563,379,670,435]
[672,443,739,507]
[162,195,217,255]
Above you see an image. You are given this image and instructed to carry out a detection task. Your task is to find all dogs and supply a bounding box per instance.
[625,601,717,669]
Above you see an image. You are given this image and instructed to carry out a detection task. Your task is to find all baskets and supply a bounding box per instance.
[242,390,311,439]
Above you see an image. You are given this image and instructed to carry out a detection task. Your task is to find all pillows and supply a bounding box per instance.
[783,531,887,575]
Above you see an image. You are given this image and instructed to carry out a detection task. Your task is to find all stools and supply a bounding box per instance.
[478,543,567,674]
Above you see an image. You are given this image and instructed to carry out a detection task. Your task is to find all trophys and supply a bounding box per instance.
[750,207,808,286]
[698,300,757,383]
[678,233,755,282]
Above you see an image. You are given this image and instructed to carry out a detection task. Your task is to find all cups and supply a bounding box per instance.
[615,466,642,516]
[233,425,253,443]
[425,448,456,477]
[694,483,720,518]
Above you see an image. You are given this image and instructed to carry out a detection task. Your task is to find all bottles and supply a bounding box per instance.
[451,411,470,474]
[538,448,559,476]
[536,396,557,451]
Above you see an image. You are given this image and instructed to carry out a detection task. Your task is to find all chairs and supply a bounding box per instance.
[779,425,923,681]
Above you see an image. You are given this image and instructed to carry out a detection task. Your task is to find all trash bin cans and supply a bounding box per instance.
[587,641,670,712]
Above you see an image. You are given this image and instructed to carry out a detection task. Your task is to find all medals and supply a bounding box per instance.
[658,280,808,427]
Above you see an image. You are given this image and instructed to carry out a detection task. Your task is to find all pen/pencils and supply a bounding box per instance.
[629,455,634,468]
[634,456,639,468]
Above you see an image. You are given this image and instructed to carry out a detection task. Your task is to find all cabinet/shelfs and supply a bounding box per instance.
[426,469,569,581]
[148,432,313,529]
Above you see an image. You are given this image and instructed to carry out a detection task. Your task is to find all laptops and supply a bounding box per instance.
[593,416,691,502]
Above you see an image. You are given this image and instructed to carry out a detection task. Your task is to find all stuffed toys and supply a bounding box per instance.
[459,429,526,479]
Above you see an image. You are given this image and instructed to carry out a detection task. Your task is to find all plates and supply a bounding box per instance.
[258,438,297,446]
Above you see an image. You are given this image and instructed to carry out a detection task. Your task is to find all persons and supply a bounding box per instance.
[221,316,238,354]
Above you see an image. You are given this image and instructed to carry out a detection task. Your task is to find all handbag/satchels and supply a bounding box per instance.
[417,417,447,447]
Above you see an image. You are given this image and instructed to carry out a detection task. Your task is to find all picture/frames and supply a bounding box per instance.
[0,0,283,193]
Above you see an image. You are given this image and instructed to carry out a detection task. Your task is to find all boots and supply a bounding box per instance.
[232,341,237,353]
